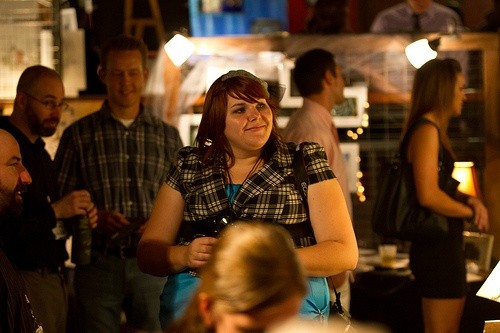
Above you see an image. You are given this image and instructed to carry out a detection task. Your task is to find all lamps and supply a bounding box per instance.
[406,35,444,69]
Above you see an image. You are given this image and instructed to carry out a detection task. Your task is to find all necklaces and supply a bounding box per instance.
[232,155,261,205]
[24,293,44,333]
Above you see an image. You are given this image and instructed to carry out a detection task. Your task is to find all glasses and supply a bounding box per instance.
[26,92,69,112]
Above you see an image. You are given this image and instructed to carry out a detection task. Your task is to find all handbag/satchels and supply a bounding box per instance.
[370,120,463,242]
[328,302,354,333]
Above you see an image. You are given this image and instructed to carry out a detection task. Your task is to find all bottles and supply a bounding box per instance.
[71,210,91,265]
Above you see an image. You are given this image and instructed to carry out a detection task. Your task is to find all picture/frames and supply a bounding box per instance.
[180,114,202,147]
[278,58,304,107]
[331,86,368,128]
[339,143,360,192]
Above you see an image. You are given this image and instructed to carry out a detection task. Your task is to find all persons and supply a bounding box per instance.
[169,220,308,333]
[398,58,489,333]
[370,0,470,171]
[0,128,70,333]
[138,70,358,333]
[0,65,98,333]
[281,48,349,314]
[55,35,184,333]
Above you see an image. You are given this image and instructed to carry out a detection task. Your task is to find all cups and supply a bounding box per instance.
[379,245,397,261]
